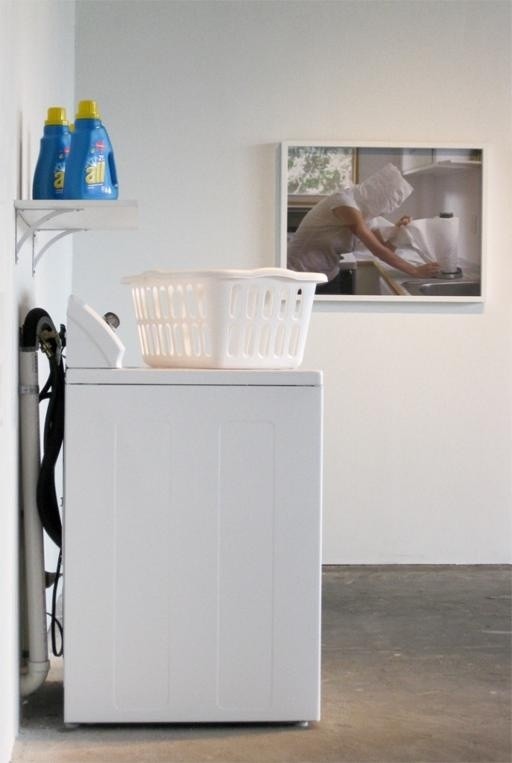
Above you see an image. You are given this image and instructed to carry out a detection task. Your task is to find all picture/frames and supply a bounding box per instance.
[280,141,487,304]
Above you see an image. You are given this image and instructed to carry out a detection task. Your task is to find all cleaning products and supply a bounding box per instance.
[63,100,119,200]
[32,107,71,199]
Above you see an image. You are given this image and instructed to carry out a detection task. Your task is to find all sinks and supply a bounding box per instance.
[403,277,484,300]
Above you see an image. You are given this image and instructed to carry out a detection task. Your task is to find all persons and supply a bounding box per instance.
[287,162,441,294]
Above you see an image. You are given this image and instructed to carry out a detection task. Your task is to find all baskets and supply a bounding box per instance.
[124,269,327,369]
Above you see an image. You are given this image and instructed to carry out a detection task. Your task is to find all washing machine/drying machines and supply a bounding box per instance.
[59,295,325,730]
